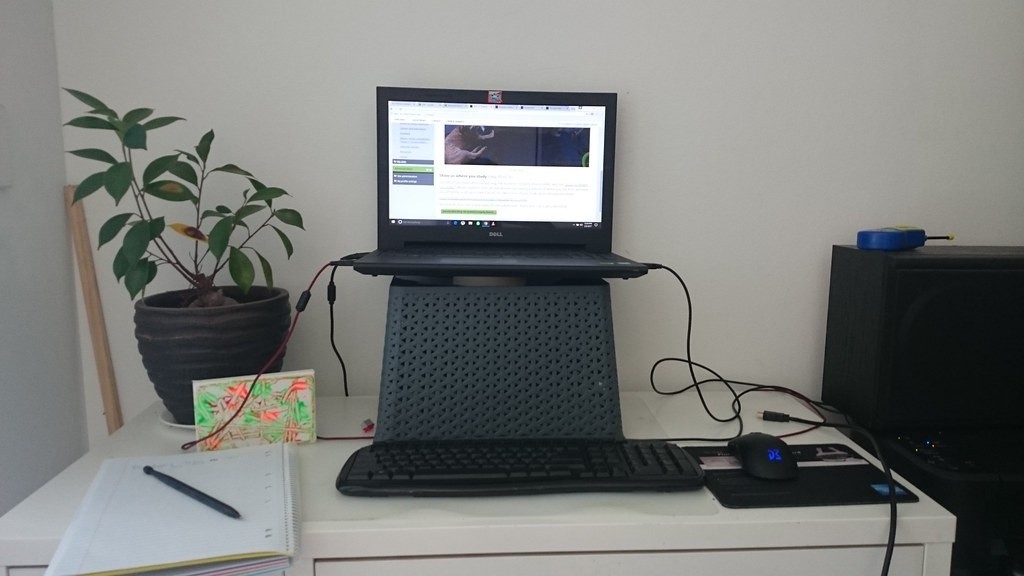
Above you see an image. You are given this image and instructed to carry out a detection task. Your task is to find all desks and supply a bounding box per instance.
[0,389,958,576]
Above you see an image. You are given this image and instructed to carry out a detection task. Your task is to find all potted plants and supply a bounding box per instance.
[60,86,308,429]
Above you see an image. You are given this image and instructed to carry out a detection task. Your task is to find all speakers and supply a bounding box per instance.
[822,244,1024,429]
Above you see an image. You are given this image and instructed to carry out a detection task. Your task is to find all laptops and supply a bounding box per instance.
[352,86,650,278]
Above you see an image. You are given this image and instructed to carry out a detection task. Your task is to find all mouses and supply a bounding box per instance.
[727,431,800,481]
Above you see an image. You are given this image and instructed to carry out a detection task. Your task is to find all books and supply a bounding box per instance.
[44,441,303,576]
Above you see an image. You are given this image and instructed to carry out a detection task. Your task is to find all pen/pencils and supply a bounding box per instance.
[143,465,243,519]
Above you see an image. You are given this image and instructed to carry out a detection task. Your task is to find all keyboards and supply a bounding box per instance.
[334,436,703,496]
